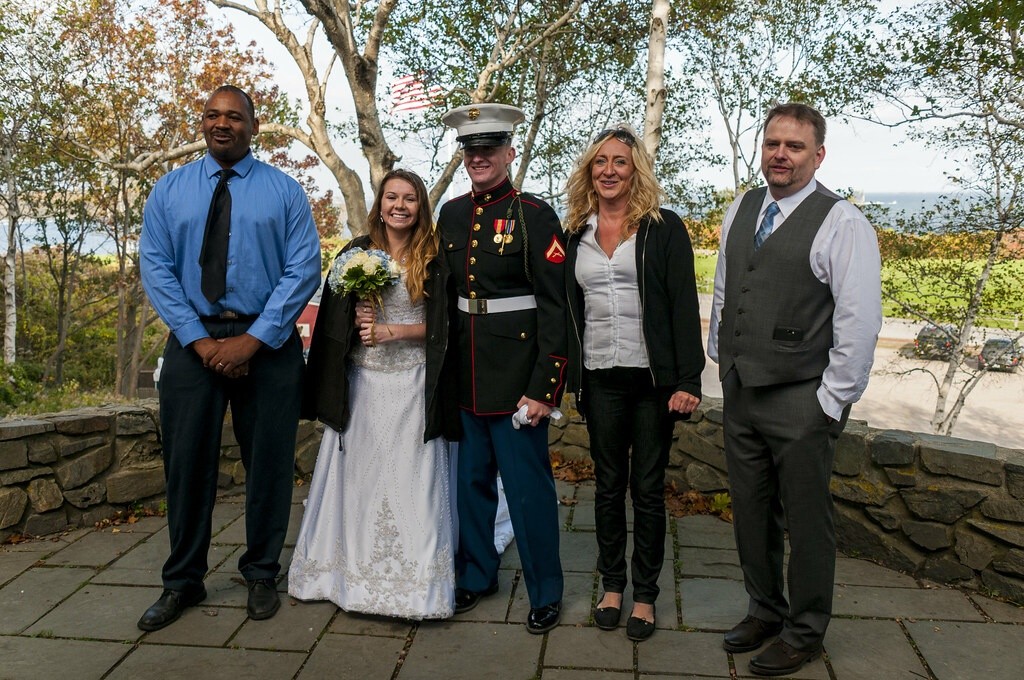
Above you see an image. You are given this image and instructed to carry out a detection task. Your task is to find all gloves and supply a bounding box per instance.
[511,403,562,430]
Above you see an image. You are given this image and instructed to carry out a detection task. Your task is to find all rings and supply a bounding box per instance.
[218,363,226,368]
[364,307,367,313]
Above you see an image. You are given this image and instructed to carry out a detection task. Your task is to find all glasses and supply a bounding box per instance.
[593,130,637,148]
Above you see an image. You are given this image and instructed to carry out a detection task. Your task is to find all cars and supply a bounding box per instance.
[978,337,1023,374]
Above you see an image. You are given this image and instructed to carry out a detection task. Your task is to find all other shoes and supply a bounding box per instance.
[626,603,657,642]
[594,593,623,631]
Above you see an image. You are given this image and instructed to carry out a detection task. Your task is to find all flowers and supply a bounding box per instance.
[328,246,402,349]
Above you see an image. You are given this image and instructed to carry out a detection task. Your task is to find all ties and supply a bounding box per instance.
[754,202,780,247]
[197,170,237,306]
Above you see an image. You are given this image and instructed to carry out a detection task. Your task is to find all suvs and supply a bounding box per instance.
[914,323,958,362]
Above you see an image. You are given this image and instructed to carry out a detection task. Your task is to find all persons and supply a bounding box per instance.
[136,86,321,631]
[287,169,460,620]
[707,103,883,675]
[433,102,567,633]
[560,123,705,641]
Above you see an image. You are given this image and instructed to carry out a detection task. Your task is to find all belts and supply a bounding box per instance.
[455,295,538,314]
[201,310,258,322]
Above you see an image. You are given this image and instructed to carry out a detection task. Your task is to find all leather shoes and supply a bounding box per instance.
[749,638,821,676]
[245,579,280,619]
[137,589,208,632]
[524,600,562,634]
[723,615,784,652]
[453,588,498,614]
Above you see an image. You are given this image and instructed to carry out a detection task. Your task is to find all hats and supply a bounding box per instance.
[440,103,526,150]
